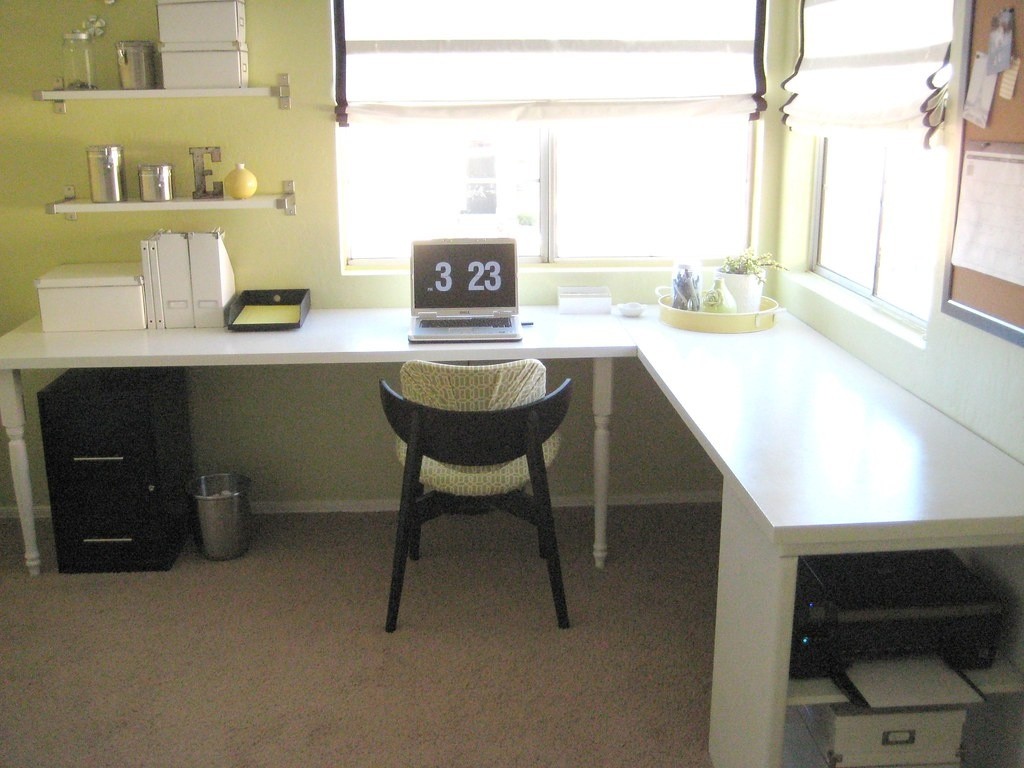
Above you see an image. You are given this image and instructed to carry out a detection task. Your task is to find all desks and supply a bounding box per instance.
[0,303,1024,768]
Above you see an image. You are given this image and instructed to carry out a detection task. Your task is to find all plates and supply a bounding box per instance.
[617,302,646,318]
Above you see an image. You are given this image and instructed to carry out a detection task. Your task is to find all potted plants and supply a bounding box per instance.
[714,252,790,315]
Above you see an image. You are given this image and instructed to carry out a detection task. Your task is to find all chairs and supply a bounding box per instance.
[379,359,571,632]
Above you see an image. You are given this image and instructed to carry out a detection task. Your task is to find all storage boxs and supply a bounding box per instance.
[34,263,147,333]
[797,704,967,768]
[556,285,612,314]
[155,1,249,90]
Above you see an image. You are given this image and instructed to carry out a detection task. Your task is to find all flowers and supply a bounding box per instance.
[704,288,723,309]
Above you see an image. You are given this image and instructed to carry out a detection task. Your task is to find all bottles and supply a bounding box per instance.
[61,32,97,91]
[224,163,259,199]
[703,277,737,315]
[672,258,702,312]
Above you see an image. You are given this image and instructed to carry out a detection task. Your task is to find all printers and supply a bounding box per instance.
[789,547,1006,709]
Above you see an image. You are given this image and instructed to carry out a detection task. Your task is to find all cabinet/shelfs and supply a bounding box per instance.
[40,72,298,217]
[36,367,194,575]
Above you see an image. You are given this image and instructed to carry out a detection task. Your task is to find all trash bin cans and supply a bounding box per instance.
[185,473,252,561]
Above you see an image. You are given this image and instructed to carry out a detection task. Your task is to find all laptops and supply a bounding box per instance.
[408,237,523,342]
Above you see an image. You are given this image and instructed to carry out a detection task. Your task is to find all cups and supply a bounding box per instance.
[85,144,125,203]
[114,39,156,90]
[137,163,173,202]
